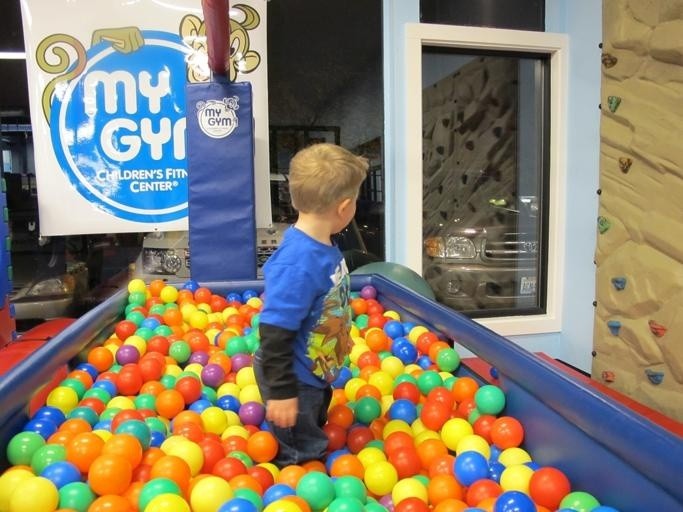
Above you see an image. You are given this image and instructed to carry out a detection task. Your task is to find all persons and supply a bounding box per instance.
[253,141,370,468]
[253,142,370,468]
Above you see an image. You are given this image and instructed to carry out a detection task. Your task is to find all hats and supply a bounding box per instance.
[134,172,367,282]
[422,165,541,307]
[8,172,89,320]
